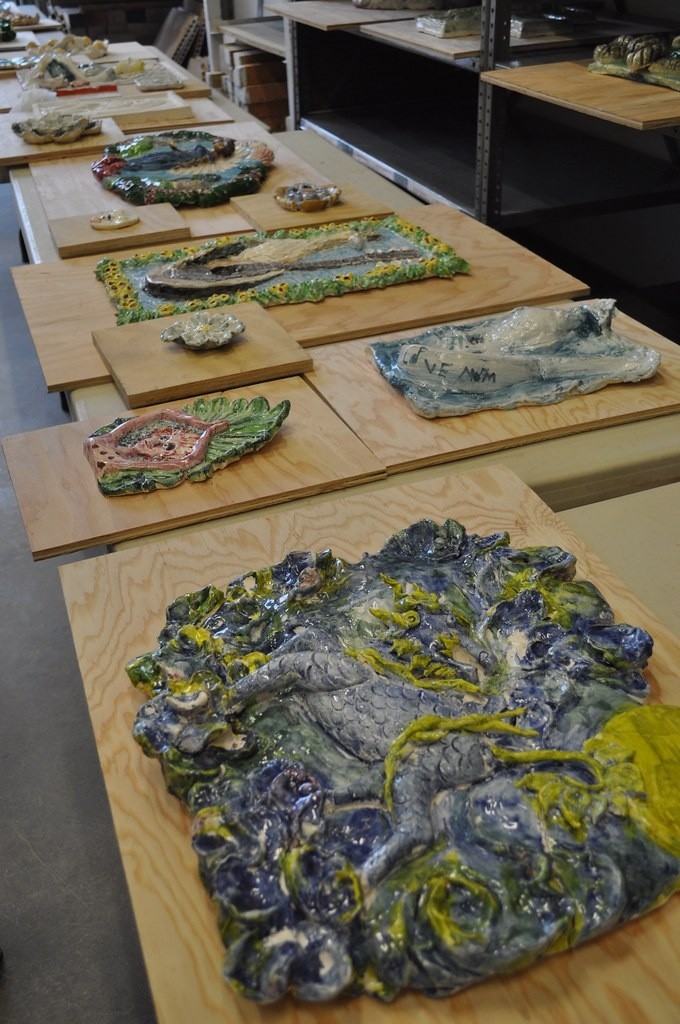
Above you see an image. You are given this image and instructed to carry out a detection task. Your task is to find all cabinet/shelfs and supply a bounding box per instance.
[201,0,680,306]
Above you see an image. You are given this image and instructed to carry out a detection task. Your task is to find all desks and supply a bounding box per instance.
[0,4,680,651]
